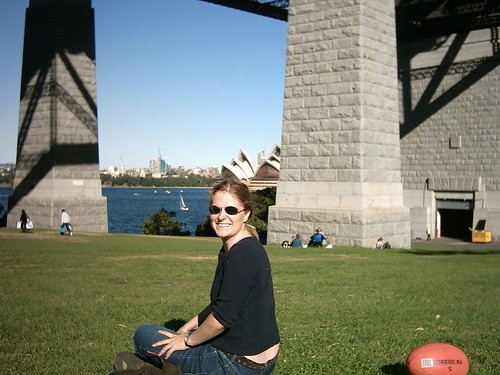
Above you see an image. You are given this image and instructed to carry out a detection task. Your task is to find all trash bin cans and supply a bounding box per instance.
[472,230,492,243]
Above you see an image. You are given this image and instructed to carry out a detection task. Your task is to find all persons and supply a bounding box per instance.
[290,227,327,247]
[426,227,431,241]
[376,238,392,249]
[114,179,280,375]
[59,209,73,236]
[17,209,33,233]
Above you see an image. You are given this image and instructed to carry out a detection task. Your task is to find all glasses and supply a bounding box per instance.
[208,205,250,215]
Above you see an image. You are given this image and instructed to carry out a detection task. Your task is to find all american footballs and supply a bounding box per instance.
[407,343,469,374]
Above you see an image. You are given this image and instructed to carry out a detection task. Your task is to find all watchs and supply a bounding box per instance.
[184,333,194,348]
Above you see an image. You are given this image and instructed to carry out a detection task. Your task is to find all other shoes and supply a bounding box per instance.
[114,352,160,371]
